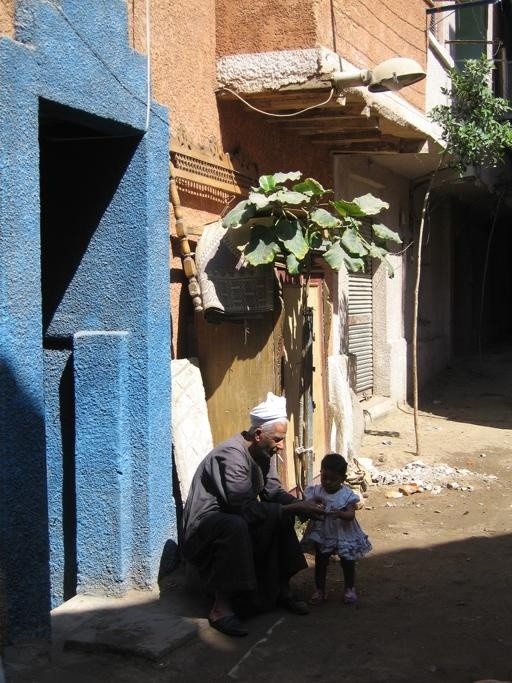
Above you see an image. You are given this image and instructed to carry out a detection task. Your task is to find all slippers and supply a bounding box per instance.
[208,612,250,637]
[287,598,310,615]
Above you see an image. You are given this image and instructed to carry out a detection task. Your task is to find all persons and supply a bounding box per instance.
[176,391,327,636]
[297,452,373,603]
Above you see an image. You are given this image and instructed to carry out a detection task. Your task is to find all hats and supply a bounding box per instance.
[248,390,288,424]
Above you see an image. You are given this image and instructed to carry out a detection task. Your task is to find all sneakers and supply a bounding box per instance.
[342,586,358,605]
[308,584,330,608]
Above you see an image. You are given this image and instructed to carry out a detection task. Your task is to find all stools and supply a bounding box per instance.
[184,565,280,596]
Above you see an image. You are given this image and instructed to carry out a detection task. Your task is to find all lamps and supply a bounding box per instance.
[332,57,426,92]
